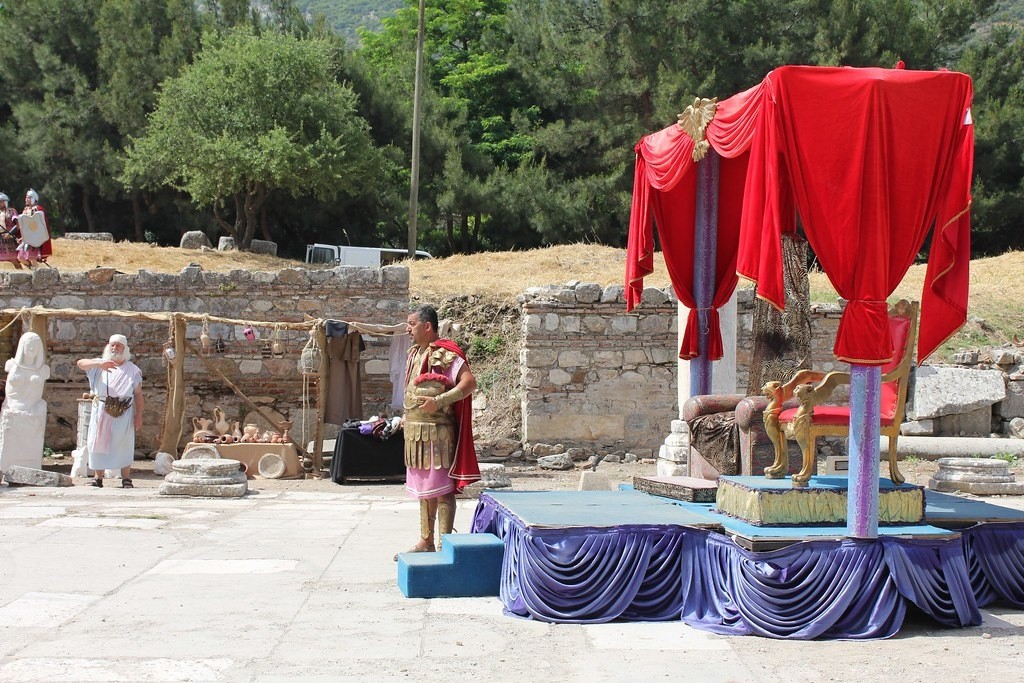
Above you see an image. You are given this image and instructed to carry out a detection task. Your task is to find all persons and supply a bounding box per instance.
[392,304,476,562]
[4,332,50,410]
[77,334,143,488]
[16,189,51,269]
[0,192,21,270]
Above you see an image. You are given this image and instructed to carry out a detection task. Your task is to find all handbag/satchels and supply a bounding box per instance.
[104,396,131,418]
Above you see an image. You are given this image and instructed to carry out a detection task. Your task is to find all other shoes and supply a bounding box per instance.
[393,545,435,561]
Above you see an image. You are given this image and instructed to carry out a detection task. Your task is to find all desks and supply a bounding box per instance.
[331,427,406,485]
[180,442,305,479]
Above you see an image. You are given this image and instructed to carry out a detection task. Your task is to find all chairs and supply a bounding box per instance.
[684,232,818,478]
[761,299,920,487]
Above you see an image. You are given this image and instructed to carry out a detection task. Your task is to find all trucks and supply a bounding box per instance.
[305,243,433,270]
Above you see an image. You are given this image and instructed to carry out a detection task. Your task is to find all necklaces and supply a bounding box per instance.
[12,357,43,370]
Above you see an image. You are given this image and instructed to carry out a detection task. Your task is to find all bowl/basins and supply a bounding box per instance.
[258,453,286,478]
[240,463,249,478]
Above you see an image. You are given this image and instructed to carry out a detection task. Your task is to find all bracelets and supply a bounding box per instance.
[435,395,444,408]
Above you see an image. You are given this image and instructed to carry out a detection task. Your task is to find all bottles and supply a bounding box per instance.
[193,408,291,443]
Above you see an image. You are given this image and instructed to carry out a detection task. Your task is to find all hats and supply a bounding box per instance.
[26,188,38,204]
[0,191,9,201]
[108,334,127,348]
[403,373,457,414]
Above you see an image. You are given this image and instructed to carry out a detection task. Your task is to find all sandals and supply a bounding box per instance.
[84,477,103,487]
[122,479,134,488]
